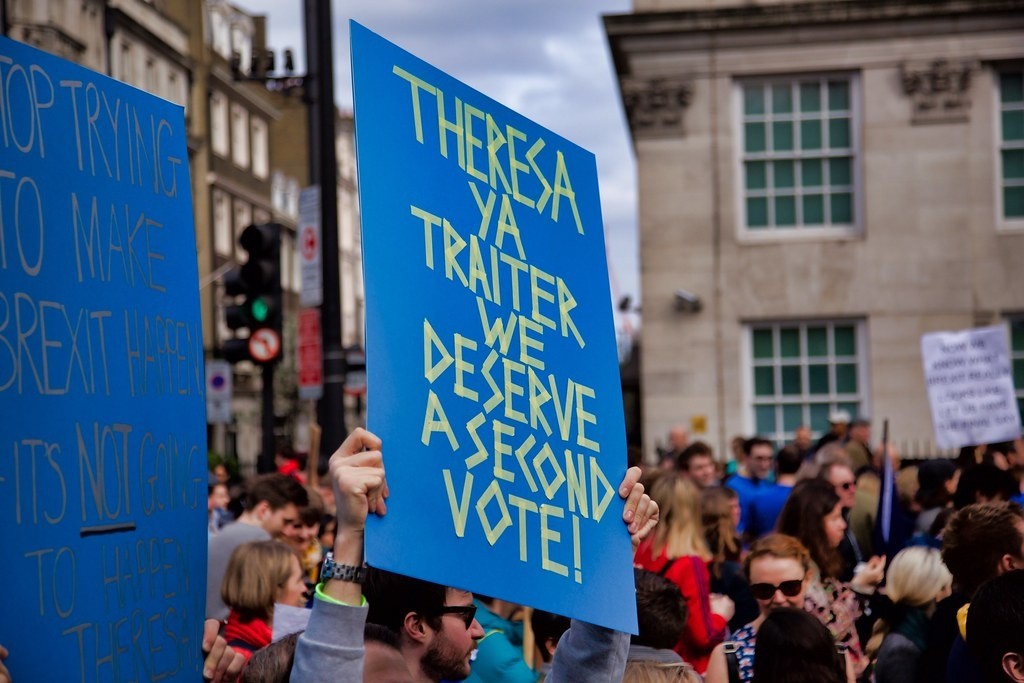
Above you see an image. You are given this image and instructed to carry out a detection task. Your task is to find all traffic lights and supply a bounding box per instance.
[218,266,252,366]
[238,222,283,327]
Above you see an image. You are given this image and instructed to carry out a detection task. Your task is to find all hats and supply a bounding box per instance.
[827,410,851,425]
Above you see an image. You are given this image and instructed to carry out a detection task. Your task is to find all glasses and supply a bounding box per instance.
[750,573,807,601]
[832,480,857,490]
[411,604,477,630]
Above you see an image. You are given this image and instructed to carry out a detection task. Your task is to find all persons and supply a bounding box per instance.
[361,563,484,683]
[463,592,542,682]
[289,428,659,683]
[528,414,1024,683]
[0,461,335,683]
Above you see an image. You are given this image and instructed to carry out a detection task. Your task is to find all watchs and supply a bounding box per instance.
[320,552,368,583]
